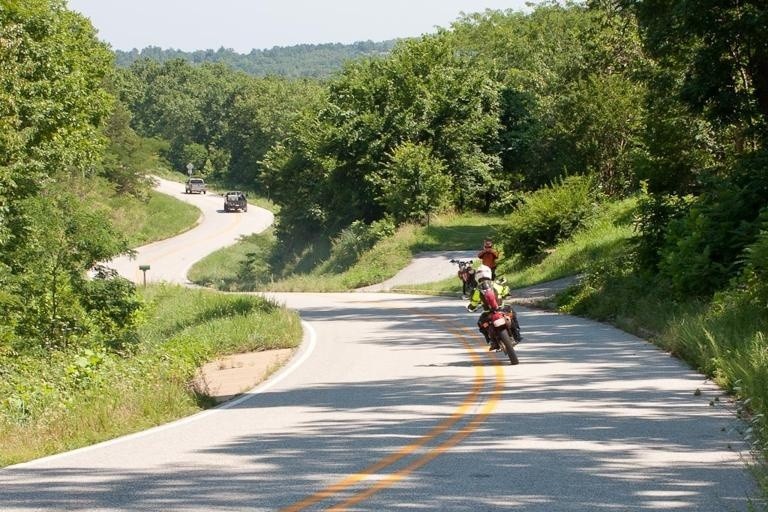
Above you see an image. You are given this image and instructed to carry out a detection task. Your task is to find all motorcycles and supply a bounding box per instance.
[449,257,523,365]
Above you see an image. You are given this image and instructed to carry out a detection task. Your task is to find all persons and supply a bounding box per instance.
[478,241,498,281]
[466,265,522,351]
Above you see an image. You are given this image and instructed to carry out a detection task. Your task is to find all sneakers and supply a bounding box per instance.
[487,334,524,352]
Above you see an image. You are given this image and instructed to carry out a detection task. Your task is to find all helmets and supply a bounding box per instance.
[483,242,493,250]
[474,266,493,282]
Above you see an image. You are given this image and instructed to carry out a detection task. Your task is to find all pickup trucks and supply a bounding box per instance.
[184,177,207,194]
[224,191,248,212]
